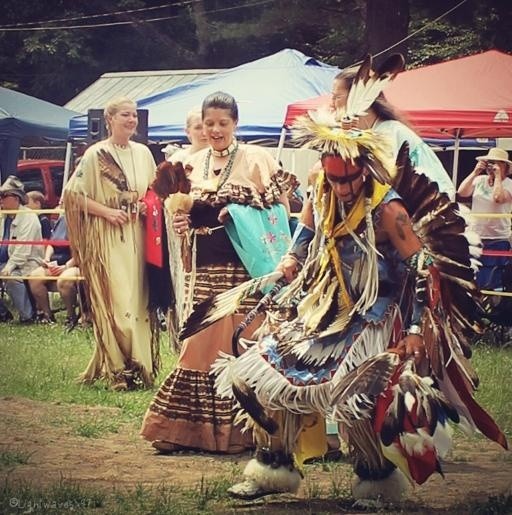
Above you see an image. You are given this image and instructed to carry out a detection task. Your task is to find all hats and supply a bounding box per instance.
[474,146,512,176]
[0,174,29,205]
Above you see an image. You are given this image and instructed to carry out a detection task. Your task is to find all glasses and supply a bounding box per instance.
[0,191,14,199]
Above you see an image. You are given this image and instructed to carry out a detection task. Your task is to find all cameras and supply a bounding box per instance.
[486,161,496,170]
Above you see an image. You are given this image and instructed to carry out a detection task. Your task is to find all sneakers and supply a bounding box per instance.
[227,481,280,501]
[62,315,78,333]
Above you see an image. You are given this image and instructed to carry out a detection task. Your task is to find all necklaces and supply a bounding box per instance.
[110,140,131,150]
[202,138,240,189]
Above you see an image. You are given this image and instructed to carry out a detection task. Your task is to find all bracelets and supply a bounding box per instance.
[405,326,425,336]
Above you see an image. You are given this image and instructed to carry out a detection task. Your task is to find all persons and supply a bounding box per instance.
[141,89,293,454]
[166,110,205,164]
[456,147,511,347]
[27,196,87,330]
[217,122,447,511]
[61,97,160,391]
[25,191,51,246]
[331,60,459,204]
[1,173,41,322]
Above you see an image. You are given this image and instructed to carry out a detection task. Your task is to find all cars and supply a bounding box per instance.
[15,155,65,209]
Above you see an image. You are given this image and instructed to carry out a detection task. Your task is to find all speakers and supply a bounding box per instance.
[87,109,148,146]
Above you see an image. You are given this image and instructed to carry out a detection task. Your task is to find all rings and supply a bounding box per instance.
[413,352,420,353]
